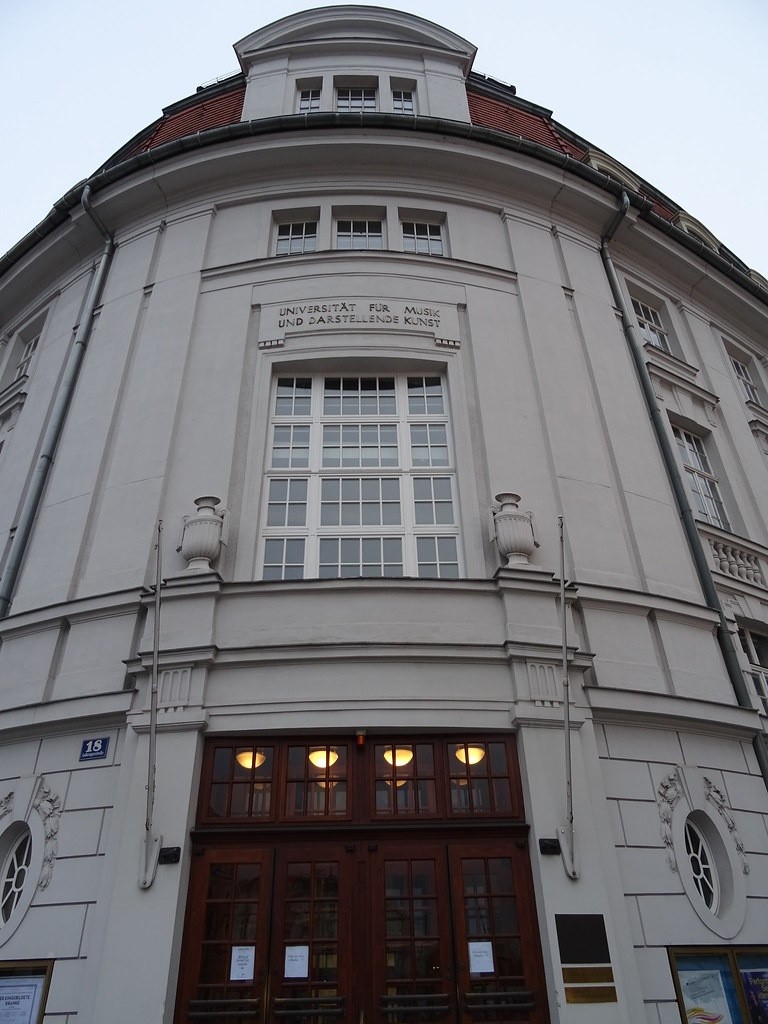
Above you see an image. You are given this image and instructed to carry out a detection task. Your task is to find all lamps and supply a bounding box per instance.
[455,744,485,764]
[237,749,265,769]
[310,749,339,768]
[383,746,413,766]
